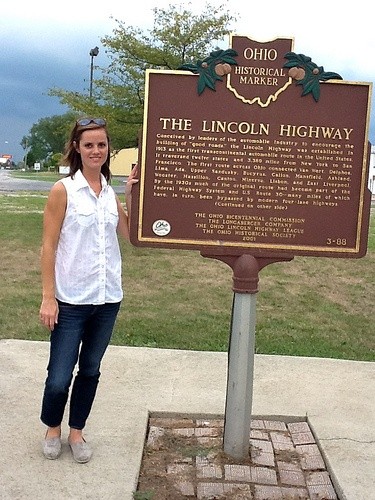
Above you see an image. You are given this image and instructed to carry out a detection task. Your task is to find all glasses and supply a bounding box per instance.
[75,118,106,133]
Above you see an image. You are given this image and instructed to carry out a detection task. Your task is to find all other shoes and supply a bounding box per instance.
[43,428,61,459]
[68,435,91,464]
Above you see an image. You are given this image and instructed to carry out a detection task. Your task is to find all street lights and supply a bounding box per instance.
[5,137,28,169]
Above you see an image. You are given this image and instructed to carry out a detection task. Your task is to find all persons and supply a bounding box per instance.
[38,118,139,463]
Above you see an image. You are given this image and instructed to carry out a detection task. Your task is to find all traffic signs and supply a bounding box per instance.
[130,31,373,294]
